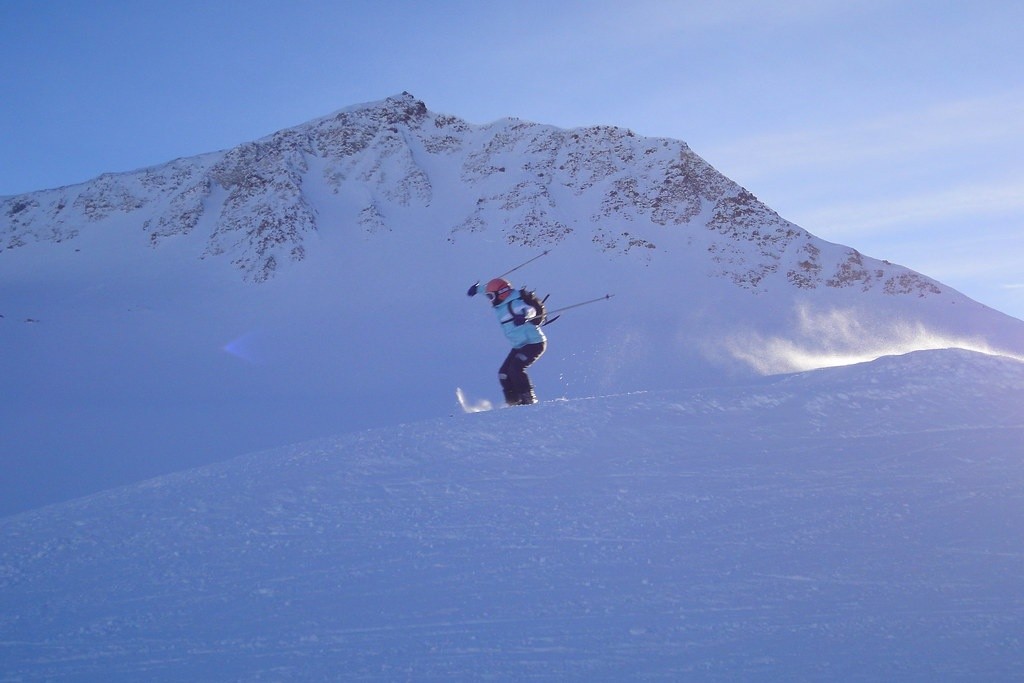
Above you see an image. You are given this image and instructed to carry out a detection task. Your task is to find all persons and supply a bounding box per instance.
[468,277,548,404]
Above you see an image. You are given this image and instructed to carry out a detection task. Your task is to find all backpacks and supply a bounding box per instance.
[508,290,546,326]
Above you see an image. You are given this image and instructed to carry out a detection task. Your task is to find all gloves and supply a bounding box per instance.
[467,283,479,296]
[514,316,525,326]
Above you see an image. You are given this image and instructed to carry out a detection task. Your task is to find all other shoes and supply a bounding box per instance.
[519,392,538,405]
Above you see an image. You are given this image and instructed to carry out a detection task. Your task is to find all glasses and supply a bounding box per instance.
[485,292,496,302]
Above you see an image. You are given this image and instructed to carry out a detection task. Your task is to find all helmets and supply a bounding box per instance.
[487,279,509,300]
